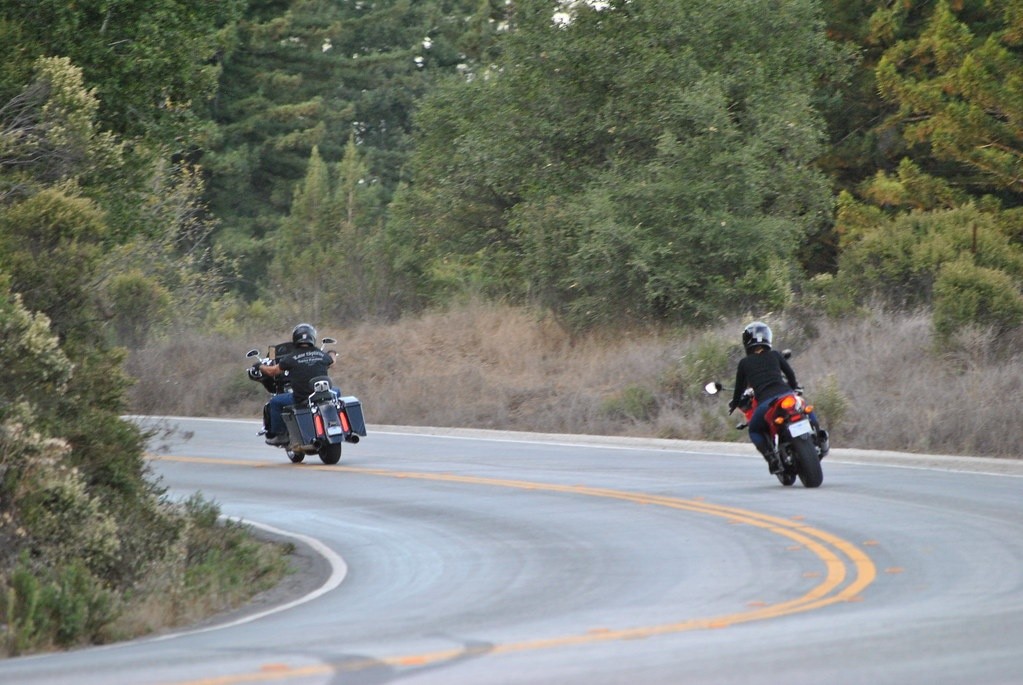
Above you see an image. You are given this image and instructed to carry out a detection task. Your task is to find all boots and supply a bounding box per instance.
[757,441,778,472]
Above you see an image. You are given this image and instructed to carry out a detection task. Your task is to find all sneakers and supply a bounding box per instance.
[264,433,290,444]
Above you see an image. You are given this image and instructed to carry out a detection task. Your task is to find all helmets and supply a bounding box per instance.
[292,323,316,347]
[742,320,772,350]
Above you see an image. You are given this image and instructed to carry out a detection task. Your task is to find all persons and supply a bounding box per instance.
[252,323,341,445]
[729,322,821,473]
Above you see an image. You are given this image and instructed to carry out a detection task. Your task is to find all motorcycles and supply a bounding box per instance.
[244,338,366,466]
[704,348,830,489]
